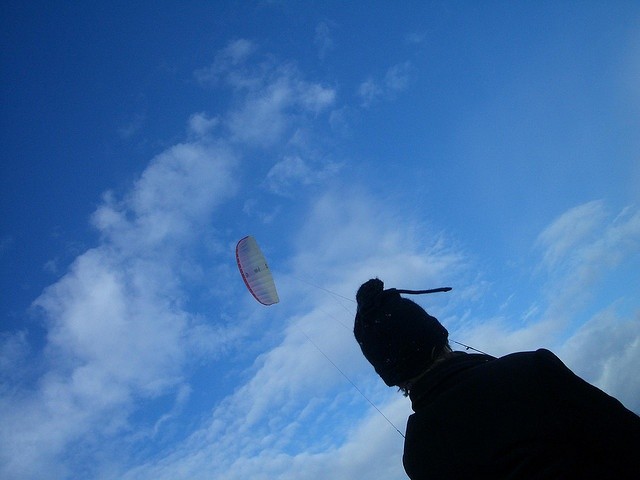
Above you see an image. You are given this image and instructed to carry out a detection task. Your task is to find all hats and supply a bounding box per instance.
[354,279,447,387]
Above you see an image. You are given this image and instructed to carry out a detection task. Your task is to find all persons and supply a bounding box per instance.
[354,278,639,480]
